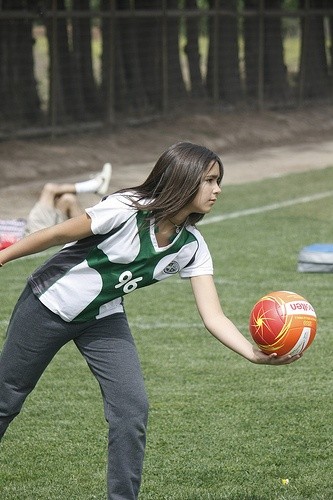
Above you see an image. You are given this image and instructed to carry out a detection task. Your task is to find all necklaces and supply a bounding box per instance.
[168,217,183,234]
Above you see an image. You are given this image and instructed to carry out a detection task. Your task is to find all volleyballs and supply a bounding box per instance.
[250,289,321,357]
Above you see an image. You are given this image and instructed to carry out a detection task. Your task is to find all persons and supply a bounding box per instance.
[0,163,112,251]
[0,141,304,500]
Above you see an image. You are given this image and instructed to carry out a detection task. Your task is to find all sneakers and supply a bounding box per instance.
[94,162,111,197]
